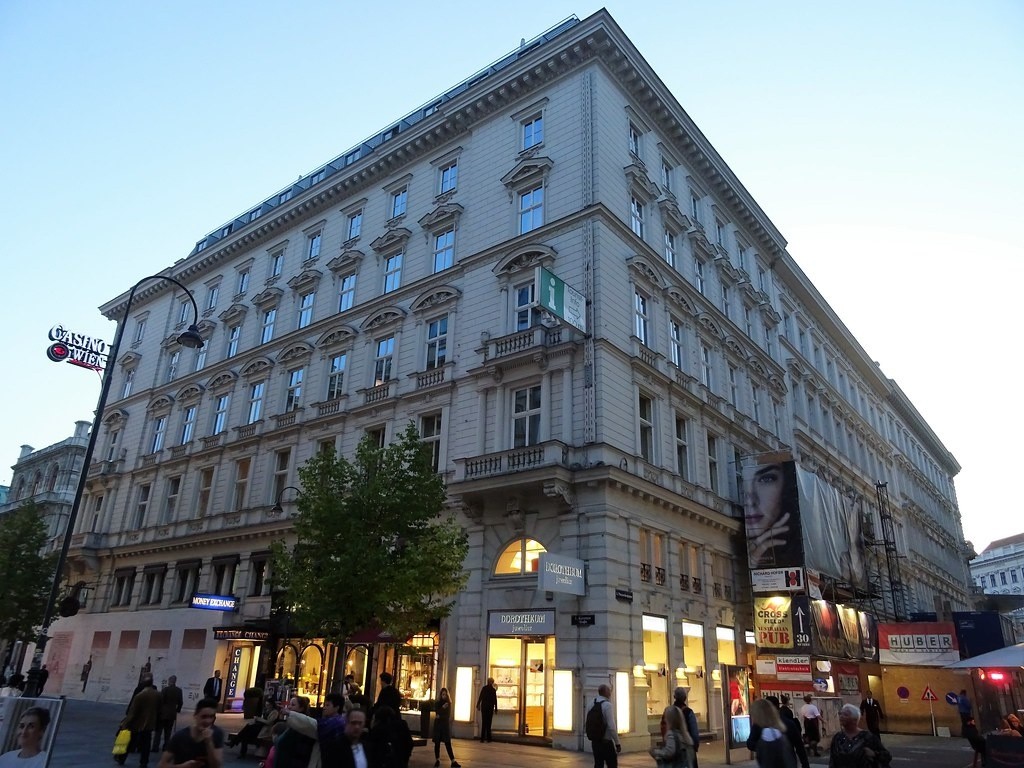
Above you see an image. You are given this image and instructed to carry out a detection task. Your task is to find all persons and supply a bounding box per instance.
[80,654,92,693]
[743,464,790,569]
[746,689,892,768]
[432,688,461,768]
[113,670,414,768]
[476,677,498,743]
[648,687,700,768]
[0,661,49,696]
[0,707,51,768]
[964,689,1024,768]
[957,689,972,739]
[586,684,621,768]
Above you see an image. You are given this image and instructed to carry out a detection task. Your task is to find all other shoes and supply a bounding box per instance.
[434,760,439,766]
[225,741,234,748]
[151,748,158,752]
[114,755,124,765]
[238,754,246,759]
[451,761,461,768]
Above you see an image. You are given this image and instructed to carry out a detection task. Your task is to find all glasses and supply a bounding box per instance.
[350,720,363,725]
[200,714,215,719]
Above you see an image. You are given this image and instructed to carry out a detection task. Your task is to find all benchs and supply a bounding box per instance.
[229,719,286,746]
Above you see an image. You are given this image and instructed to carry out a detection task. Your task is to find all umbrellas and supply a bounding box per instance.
[941,643,1024,667]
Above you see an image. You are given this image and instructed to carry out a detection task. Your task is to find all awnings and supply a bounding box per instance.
[344,619,415,644]
[213,622,338,640]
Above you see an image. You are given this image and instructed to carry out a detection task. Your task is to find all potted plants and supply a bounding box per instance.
[243,687,263,719]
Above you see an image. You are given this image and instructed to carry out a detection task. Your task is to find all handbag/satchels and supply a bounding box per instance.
[112,728,132,755]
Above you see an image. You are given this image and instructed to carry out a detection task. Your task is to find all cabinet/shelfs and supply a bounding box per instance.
[490,664,544,731]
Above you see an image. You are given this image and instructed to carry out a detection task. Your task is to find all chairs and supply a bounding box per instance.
[966,735,1024,768]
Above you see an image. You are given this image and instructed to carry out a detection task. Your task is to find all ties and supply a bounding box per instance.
[868,699,871,707]
[214,678,217,695]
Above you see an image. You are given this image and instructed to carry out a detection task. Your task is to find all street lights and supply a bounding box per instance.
[21,275,204,697]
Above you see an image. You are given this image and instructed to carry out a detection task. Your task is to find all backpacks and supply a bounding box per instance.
[586,698,608,741]
